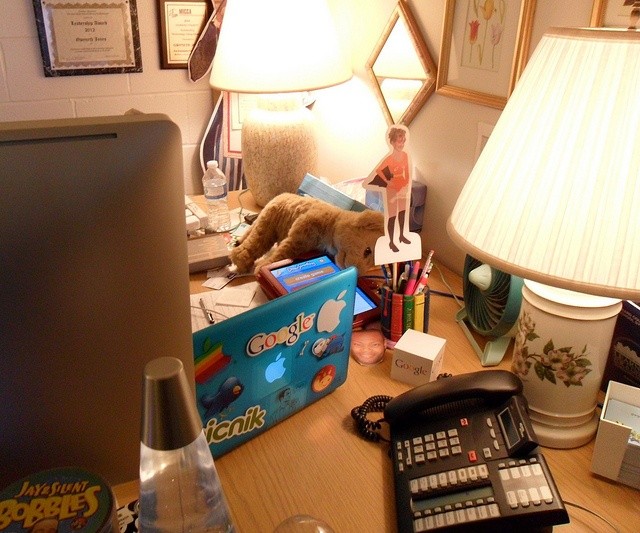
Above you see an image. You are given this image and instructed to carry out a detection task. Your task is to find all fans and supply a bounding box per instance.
[455,253,524,367]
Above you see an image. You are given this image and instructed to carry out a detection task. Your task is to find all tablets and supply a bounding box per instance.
[259,250,381,329]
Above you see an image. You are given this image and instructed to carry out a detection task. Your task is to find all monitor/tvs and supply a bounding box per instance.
[0,114,196,490]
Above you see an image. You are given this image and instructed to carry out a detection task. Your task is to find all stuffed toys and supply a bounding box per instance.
[231,193,383,279]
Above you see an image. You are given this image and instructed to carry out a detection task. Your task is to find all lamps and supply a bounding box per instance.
[209,0,353,208]
[446,27,640,451]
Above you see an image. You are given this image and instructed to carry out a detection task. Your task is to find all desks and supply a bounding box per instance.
[110,188,640,533]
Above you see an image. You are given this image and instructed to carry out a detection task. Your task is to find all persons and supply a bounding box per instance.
[377,127,411,252]
[351,329,385,364]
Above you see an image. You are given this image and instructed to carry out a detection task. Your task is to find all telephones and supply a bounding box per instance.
[351,370,569,532]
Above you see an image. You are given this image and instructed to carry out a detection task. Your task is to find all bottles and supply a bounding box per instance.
[203,159,230,229]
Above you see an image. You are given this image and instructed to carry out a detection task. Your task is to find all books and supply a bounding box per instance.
[190,282,270,333]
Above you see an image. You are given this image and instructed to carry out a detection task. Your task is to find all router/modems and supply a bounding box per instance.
[187,232,230,274]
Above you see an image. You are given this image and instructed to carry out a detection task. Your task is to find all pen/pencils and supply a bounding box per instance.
[200,298,215,324]
[382,250,434,295]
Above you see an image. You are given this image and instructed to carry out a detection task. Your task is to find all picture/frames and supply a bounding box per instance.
[366,0,437,128]
[435,0,536,111]
[589,0,640,28]
[158,0,213,69]
[33,0,143,77]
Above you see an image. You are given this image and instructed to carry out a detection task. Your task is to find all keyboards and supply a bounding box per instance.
[185,193,208,234]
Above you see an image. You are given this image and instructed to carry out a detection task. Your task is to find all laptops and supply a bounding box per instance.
[192,265,360,463]
